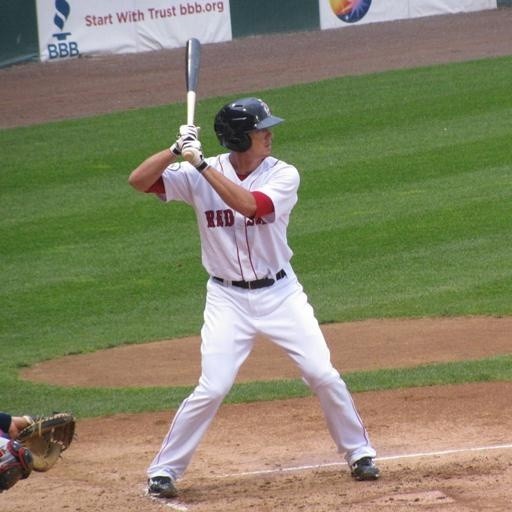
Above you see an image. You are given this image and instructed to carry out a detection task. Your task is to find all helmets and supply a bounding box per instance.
[214,97,284,152]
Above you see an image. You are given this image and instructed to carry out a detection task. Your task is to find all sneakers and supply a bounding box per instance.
[145,476,177,498]
[351,456,381,481]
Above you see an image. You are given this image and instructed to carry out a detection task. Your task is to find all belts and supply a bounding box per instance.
[213,269,286,289]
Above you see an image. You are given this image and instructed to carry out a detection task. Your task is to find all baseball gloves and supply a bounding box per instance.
[12,412,76,471]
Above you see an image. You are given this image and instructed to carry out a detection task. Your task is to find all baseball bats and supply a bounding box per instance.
[180,38,201,162]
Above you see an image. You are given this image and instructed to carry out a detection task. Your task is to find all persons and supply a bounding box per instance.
[0,413,30,440]
[128,97,380,499]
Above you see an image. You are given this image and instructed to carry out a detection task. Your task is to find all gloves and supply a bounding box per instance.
[170,124,209,173]
